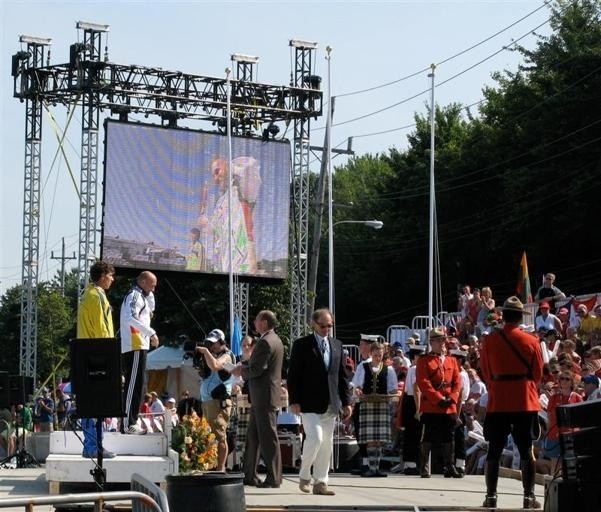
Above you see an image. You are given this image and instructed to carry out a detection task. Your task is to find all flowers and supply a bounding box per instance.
[170,407,221,476]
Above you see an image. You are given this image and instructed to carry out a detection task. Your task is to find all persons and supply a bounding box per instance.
[183,158,258,276]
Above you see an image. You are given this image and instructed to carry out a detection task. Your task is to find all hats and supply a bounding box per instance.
[427,326,452,340]
[495,295,532,317]
[393,332,421,347]
[539,302,549,309]
[168,398,176,403]
[360,333,379,342]
[406,348,423,355]
[204,328,226,343]
[559,307,569,316]
[543,329,558,336]
[581,375,599,387]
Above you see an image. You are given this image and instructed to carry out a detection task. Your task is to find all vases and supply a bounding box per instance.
[164,471,248,512]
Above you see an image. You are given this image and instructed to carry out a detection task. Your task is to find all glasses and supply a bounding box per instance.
[551,370,559,373]
[316,321,333,329]
[546,278,552,280]
[559,377,570,381]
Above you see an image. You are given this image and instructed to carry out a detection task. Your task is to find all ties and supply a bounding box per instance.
[319,340,327,359]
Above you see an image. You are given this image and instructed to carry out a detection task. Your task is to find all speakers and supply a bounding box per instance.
[555,400,601,512]
[8,375,33,404]
[71,338,126,417]
[0,371,14,407]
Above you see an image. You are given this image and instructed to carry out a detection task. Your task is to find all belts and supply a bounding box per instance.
[433,383,453,389]
[492,373,530,382]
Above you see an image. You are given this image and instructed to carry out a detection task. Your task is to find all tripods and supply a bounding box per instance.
[0,404,41,468]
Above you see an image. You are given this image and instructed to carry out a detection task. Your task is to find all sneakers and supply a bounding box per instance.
[313,482,335,496]
[523,495,542,509]
[483,493,498,508]
[240,474,261,486]
[257,479,281,489]
[351,463,420,478]
[444,467,465,478]
[120,424,148,436]
[420,464,432,478]
[298,478,311,494]
[82,446,117,459]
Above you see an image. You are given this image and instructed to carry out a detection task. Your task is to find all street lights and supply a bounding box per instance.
[309,219,390,332]
